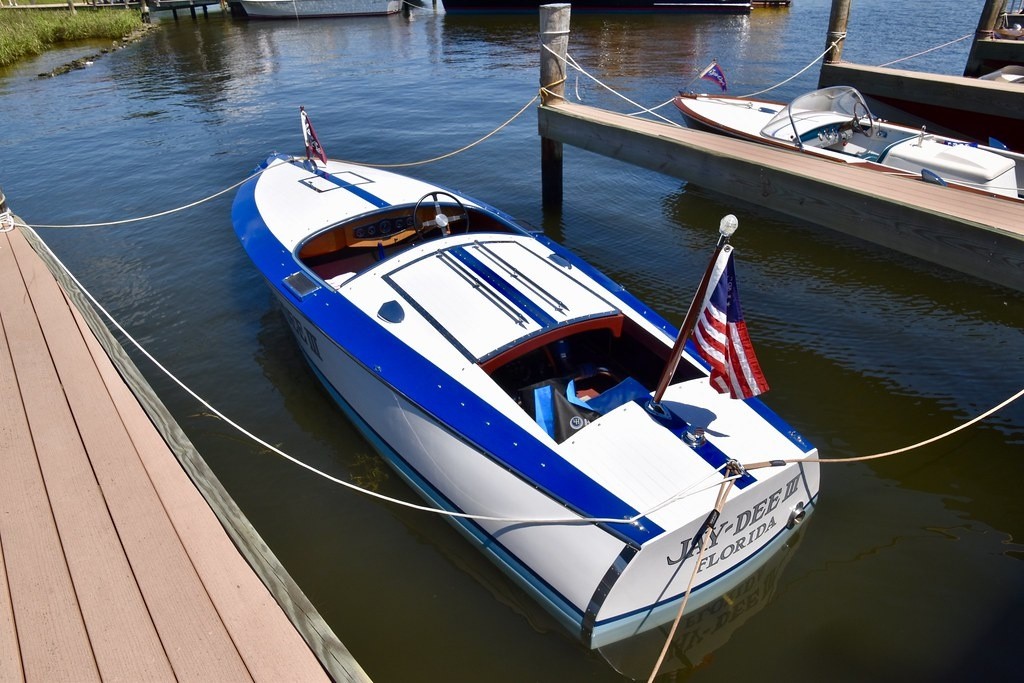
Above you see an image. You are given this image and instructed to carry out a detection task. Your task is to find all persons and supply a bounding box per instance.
[139,0,151,23]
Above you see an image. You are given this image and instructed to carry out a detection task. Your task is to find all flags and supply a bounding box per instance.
[692,246,770,399]
[701,63,727,93]
[301,113,327,165]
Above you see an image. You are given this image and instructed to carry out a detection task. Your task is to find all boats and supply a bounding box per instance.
[978,65,1024,84]
[230,105,821,652]
[671,58,1024,202]
